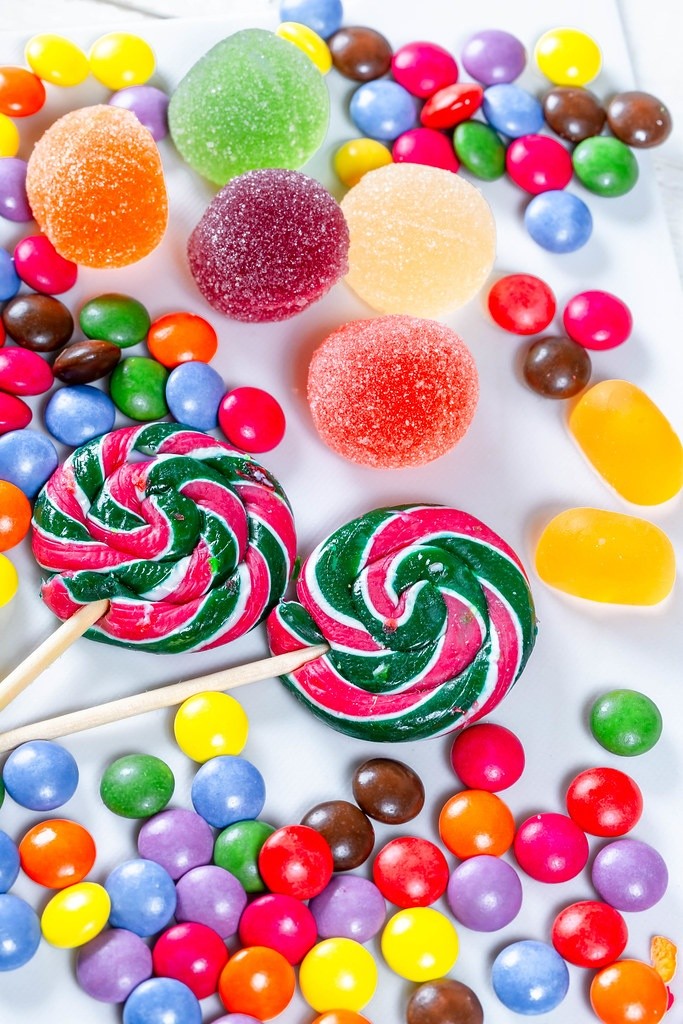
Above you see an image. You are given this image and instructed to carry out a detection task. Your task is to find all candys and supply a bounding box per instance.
[0,0,683,1024]
